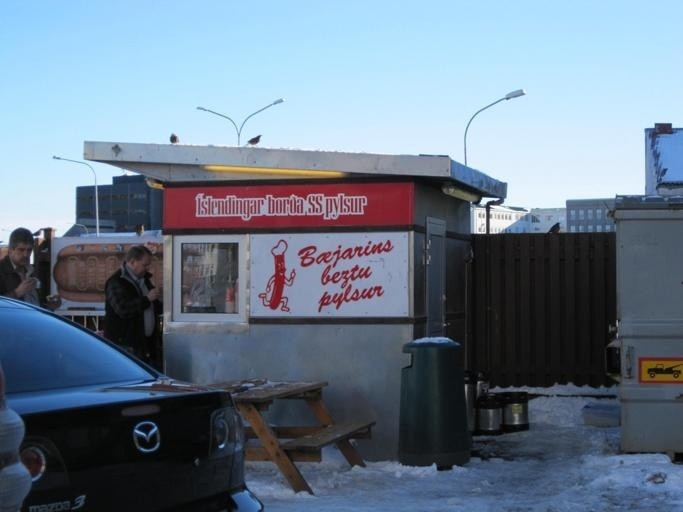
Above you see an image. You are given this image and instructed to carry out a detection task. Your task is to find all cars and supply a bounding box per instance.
[0,293,263,511]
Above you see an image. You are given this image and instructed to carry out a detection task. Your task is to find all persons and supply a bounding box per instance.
[103,244,163,362]
[0,225,63,311]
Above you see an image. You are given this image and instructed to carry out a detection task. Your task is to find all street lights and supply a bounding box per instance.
[462,89,525,165]
[196,97,285,147]
[52,155,100,236]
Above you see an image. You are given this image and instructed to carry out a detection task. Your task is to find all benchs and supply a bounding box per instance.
[276,413,377,457]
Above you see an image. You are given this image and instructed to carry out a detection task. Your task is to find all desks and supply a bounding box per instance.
[202,377,368,497]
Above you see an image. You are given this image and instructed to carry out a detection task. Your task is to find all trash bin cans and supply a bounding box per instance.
[399,335,470,466]
[479,391,529,436]
